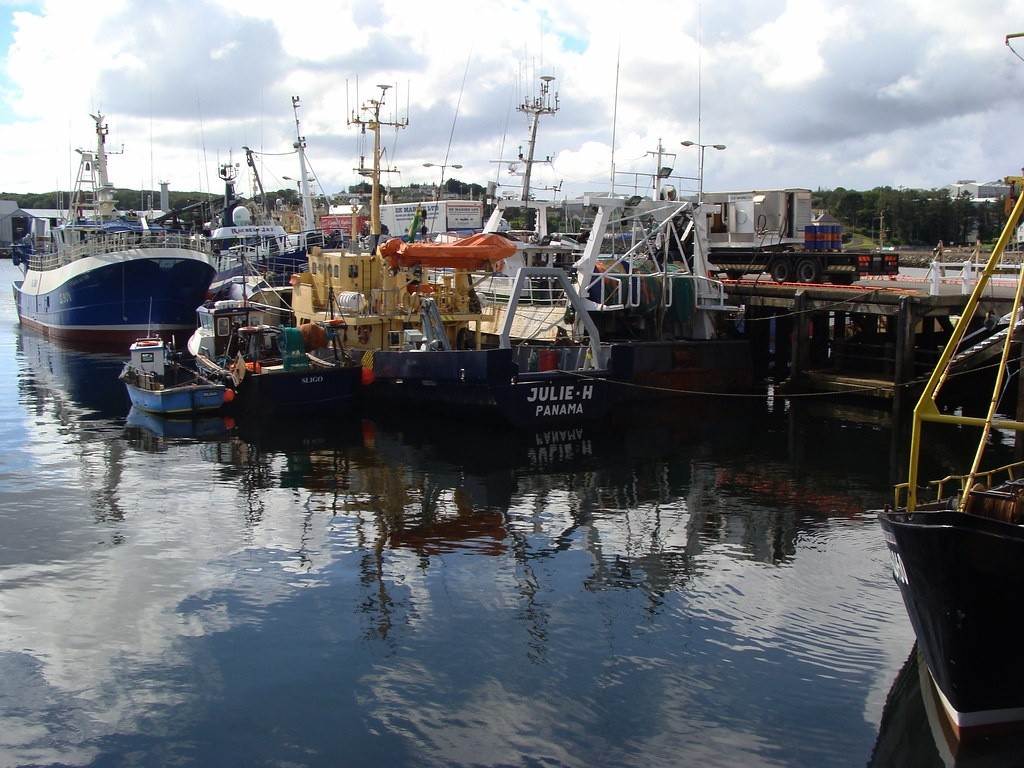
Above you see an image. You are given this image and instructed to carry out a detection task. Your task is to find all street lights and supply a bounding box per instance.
[681,140,727,203]
[421,163,463,201]
[282,176,316,209]
[843,216,855,242]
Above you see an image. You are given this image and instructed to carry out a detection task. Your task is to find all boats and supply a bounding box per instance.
[10,42,796,421]
[867,637,1023,767]
[18,326,133,412]
[869,157,1024,743]
[124,404,235,443]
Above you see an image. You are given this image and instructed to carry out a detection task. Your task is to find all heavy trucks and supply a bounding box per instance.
[646,211,899,287]
